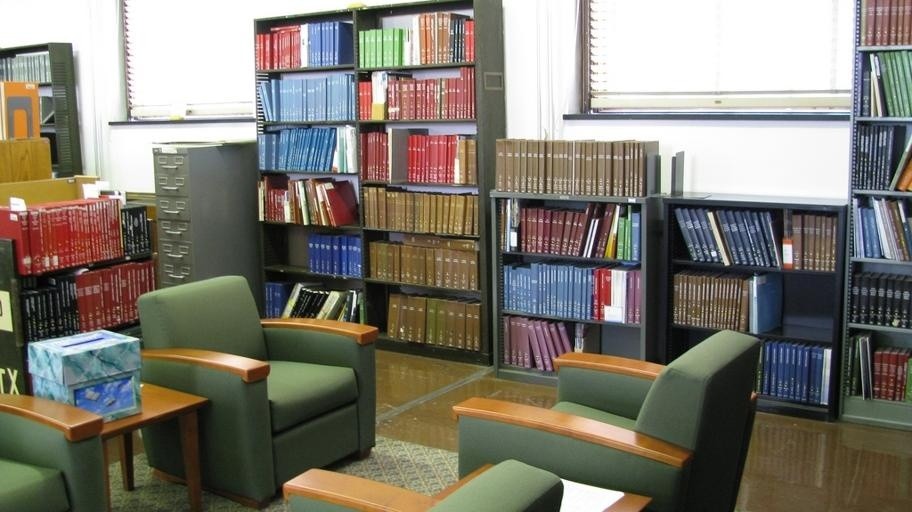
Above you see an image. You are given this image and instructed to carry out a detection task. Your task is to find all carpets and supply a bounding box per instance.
[108,432,458,512]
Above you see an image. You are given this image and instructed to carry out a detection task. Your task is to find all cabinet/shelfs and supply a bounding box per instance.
[356,0,493,367]
[253,0,368,329]
[661,192,846,422]
[839,2,912,429]
[490,189,667,389]
[1,238,154,396]
[153,137,259,292]
[2,41,84,178]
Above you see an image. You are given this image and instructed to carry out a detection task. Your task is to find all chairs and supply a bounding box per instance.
[138,275,380,512]
[0,397,107,511]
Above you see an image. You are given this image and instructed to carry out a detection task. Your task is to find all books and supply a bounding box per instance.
[1,189,156,342]
[0,55,56,139]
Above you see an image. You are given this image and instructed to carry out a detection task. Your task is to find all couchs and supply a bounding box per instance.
[451,330,760,512]
[284,458,653,511]
